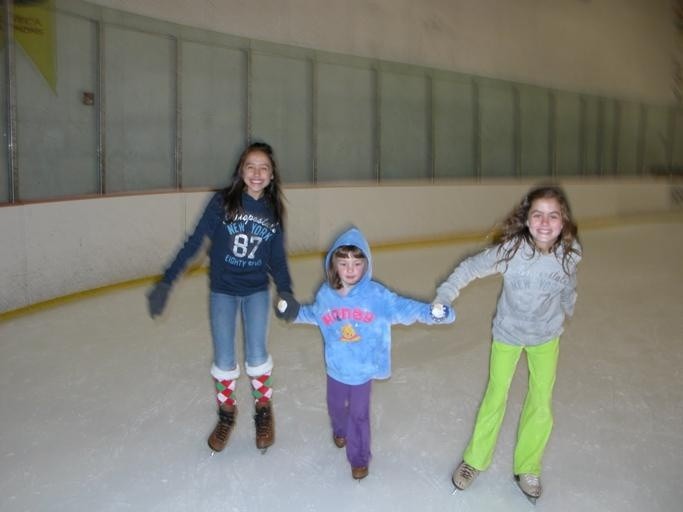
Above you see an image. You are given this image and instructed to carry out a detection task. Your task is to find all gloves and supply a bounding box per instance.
[273,291,300,323]
[431,303,456,325]
[147,281,169,316]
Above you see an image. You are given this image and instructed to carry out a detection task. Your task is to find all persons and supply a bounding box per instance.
[430,186,584,499]
[145,142,300,451]
[275,224,457,478]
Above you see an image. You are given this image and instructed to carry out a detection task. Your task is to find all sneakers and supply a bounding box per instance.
[254,400,274,449]
[333,430,346,448]
[208,404,238,452]
[452,460,480,491]
[352,464,369,479]
[515,473,542,499]
